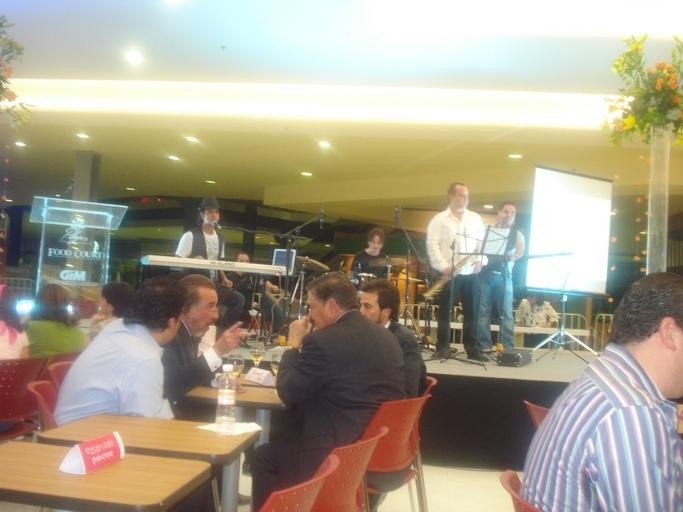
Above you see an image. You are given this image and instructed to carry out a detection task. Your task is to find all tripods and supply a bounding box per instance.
[422,247,484,367]
[417,273,457,352]
[531,294,600,357]
[536,294,590,365]
[220,226,319,345]
[482,261,518,355]
[244,277,272,343]
[397,219,435,351]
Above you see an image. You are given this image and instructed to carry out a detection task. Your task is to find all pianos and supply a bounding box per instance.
[140,255,287,280]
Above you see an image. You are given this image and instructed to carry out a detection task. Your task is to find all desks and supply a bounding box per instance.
[37,413,260,512]
[186,385,287,450]
[0,442,211,512]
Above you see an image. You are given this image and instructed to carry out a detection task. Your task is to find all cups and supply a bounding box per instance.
[250,341,265,368]
[228,351,244,378]
[271,350,285,376]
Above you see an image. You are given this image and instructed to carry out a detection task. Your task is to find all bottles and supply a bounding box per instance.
[214,364,238,429]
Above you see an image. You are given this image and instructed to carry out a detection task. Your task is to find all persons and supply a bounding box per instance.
[231,250,254,307]
[426,182,489,365]
[176,194,245,334]
[52,275,187,428]
[161,273,250,404]
[89,281,137,343]
[350,227,393,278]
[359,278,427,397]
[516,289,560,328]
[250,270,420,512]
[24,283,86,356]
[518,272,683,512]
[0,284,28,362]
[480,201,524,354]
[259,273,281,292]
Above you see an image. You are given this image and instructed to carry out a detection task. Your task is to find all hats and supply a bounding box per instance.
[196,195,219,209]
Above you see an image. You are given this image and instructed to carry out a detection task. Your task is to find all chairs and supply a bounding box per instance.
[359,393,431,512]
[407,376,437,512]
[312,424,389,512]
[500,470,540,512]
[0,358,49,441]
[523,400,550,429]
[259,454,340,512]
[26,380,58,430]
[48,361,75,392]
[33,352,81,422]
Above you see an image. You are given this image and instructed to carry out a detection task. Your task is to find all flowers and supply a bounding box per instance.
[608,32,683,145]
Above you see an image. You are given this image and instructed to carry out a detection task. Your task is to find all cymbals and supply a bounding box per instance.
[369,257,407,267]
[296,255,330,271]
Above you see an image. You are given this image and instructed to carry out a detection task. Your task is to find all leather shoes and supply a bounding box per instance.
[469,349,490,362]
[431,348,452,357]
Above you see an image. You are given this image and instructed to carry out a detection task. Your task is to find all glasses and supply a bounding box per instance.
[192,302,220,310]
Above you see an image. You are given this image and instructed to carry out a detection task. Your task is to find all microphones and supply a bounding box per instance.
[320,209,323,229]
[394,205,400,226]
[450,240,456,249]
[210,221,223,230]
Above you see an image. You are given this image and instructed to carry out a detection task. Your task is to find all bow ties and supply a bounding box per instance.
[190,335,201,344]
